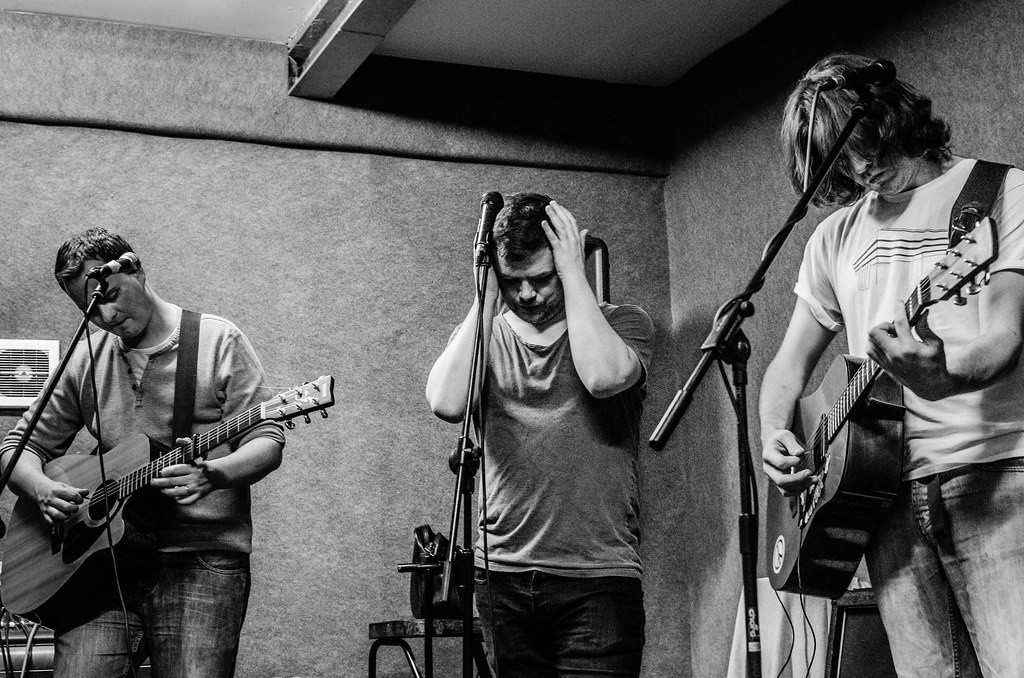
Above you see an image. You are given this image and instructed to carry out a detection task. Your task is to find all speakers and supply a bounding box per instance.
[824,591,899,678]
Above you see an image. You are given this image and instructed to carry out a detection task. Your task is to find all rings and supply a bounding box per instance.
[184,485,191,497]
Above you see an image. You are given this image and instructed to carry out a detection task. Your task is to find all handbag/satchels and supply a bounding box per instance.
[405,526,448,620]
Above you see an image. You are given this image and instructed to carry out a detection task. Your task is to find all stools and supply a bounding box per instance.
[369,616,492,678]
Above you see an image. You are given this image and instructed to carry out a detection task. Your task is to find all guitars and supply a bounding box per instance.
[1,374,335,634]
[764,215,999,601]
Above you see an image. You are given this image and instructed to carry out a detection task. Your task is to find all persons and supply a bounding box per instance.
[760,54,1024,678]
[424,191,655,678]
[0,227,285,678]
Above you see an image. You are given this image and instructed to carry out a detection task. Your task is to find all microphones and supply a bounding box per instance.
[818,58,896,91]
[474,190,503,266]
[86,252,142,280]
